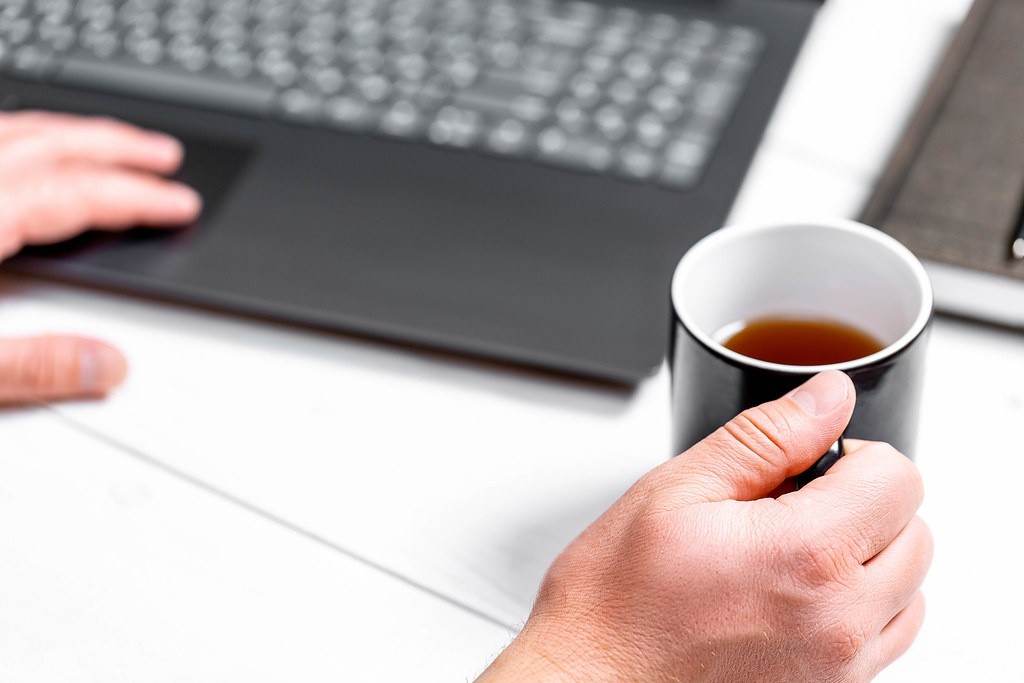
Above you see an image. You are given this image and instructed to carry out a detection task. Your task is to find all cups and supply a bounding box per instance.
[667,216,932,500]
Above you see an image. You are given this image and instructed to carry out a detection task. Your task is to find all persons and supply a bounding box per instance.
[0,109,932,683]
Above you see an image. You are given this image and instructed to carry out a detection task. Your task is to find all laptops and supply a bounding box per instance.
[0,0,827,390]
